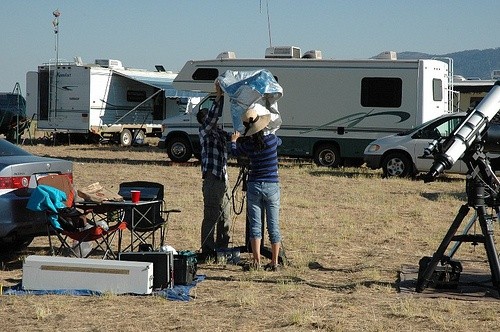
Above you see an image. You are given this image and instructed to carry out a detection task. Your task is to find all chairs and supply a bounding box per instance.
[118,181,181,253]
[36,185,126,260]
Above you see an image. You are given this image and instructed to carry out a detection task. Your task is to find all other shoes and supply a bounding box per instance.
[243,262,261,271]
[267,262,278,271]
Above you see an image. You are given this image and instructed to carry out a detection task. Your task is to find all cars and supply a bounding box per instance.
[363,110,468,178]
[0,137,75,262]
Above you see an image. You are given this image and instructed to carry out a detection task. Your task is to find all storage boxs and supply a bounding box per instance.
[77,182,123,203]
[119,251,175,290]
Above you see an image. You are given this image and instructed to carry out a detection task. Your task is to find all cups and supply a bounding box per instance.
[131,191,140,203]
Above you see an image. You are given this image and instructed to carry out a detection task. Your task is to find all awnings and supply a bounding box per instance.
[112,70,210,123]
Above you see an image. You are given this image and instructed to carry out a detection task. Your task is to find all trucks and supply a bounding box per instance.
[24,58,163,146]
[163,46,454,168]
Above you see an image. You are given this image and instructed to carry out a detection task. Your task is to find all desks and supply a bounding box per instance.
[76,200,162,260]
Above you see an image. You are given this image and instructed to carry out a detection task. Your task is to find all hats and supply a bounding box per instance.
[67,216,94,232]
[241,109,271,136]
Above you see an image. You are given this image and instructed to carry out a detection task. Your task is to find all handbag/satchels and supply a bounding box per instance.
[77,182,125,205]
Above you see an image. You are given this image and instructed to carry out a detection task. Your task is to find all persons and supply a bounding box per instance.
[231,108,283,272]
[196,80,231,257]
